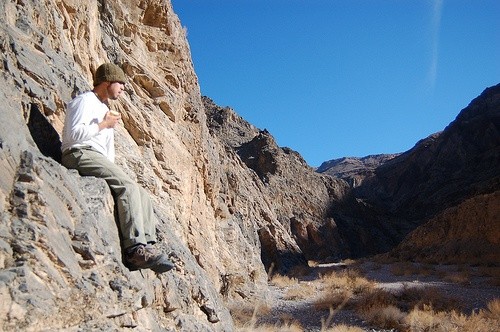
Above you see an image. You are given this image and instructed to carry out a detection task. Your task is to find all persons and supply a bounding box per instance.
[61,63,175,273]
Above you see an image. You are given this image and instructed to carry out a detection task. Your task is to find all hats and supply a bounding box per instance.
[93,62,126,87]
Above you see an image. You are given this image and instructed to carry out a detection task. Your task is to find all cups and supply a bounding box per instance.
[105,110,119,129]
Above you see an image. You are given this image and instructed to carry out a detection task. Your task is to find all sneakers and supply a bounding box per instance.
[125,245,174,274]
